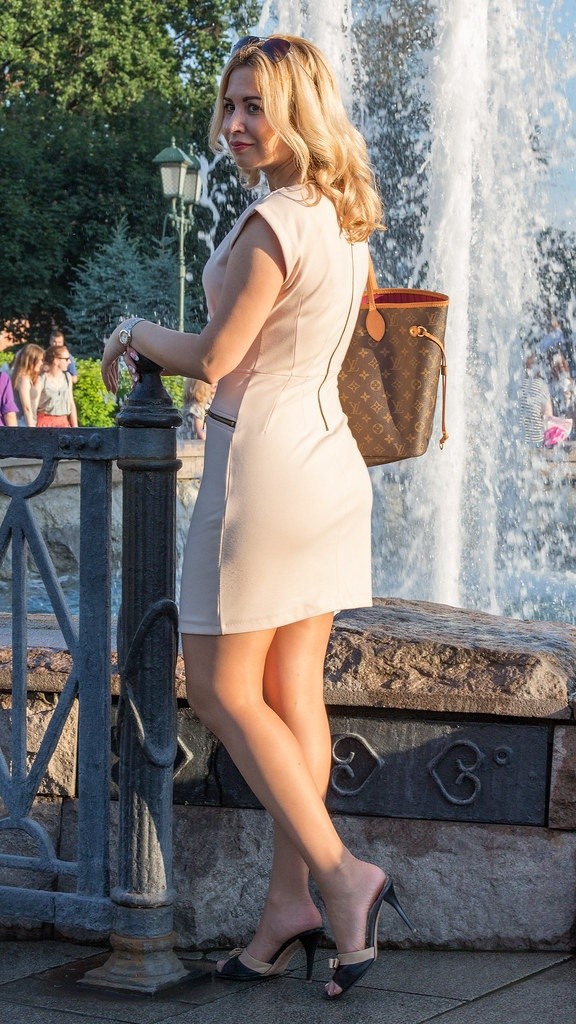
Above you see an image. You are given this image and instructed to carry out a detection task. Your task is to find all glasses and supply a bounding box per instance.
[231,36,318,87]
[56,357,71,362]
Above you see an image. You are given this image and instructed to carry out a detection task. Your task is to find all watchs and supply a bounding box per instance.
[118,318,145,346]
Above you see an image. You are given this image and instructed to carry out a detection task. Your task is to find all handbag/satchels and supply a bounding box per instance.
[337,251,451,466]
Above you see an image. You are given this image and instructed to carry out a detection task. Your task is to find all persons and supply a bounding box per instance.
[101,34,416,1001]
[0,348,23,376]
[11,345,45,427]
[36,346,78,427]
[0,372,20,426]
[522,307,576,447]
[176,378,218,440]
[49,332,78,383]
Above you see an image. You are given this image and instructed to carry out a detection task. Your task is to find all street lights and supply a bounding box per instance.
[152,136,202,333]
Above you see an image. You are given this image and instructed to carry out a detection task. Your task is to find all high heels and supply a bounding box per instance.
[214,925,326,979]
[323,874,416,1000]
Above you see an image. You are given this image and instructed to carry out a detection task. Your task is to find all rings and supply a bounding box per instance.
[123,351,126,355]
